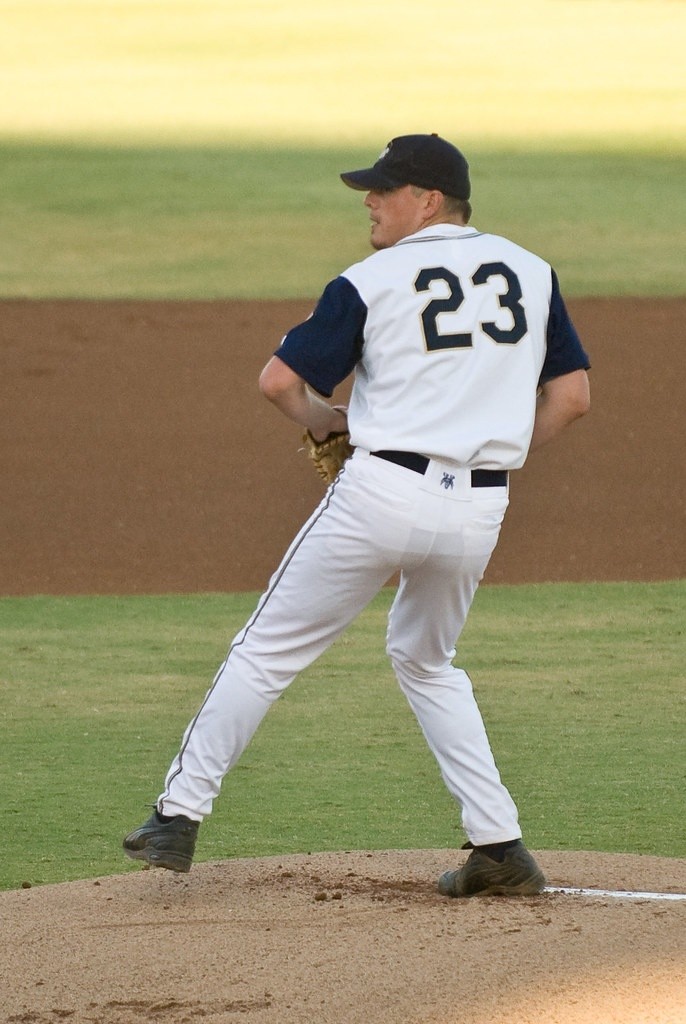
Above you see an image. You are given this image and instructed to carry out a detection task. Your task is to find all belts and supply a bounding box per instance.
[370,451,507,487]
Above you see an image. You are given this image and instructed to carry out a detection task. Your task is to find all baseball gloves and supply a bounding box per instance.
[303,405,355,485]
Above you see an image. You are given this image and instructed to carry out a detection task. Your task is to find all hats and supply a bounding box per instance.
[340,133,470,200]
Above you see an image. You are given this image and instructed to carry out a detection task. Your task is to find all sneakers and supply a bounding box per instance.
[123,805,200,873]
[438,841,546,898]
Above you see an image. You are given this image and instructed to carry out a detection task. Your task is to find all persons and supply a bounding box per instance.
[120,135,590,898]
